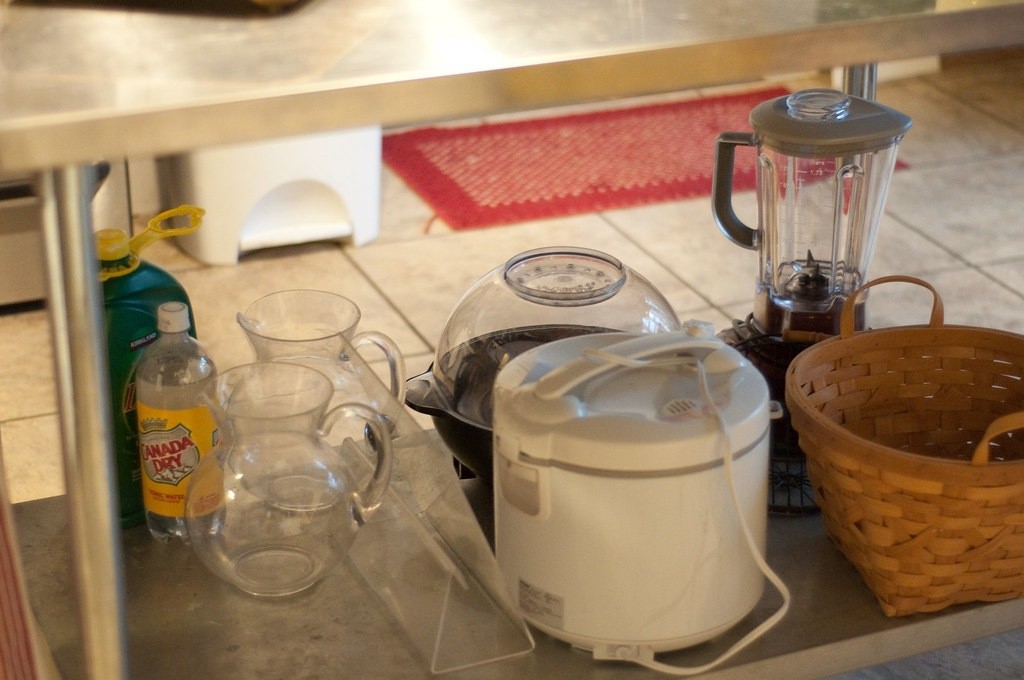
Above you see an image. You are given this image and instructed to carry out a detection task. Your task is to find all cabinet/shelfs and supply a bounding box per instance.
[0,0,1024,680]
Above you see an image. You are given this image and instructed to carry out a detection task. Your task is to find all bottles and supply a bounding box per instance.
[237,289,408,455]
[181,360,392,598]
[134,300,226,546]
[93,203,205,528]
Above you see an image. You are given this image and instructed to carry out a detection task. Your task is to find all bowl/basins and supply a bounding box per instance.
[397,246,685,494]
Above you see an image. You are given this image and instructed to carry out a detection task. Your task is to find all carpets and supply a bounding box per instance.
[382,82,910,238]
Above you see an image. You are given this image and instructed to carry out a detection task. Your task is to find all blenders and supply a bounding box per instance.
[709,88,913,518]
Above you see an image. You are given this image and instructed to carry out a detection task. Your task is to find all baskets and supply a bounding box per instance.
[783,275,1023,618]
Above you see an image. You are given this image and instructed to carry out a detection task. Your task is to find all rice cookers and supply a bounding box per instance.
[484,322,794,675]
